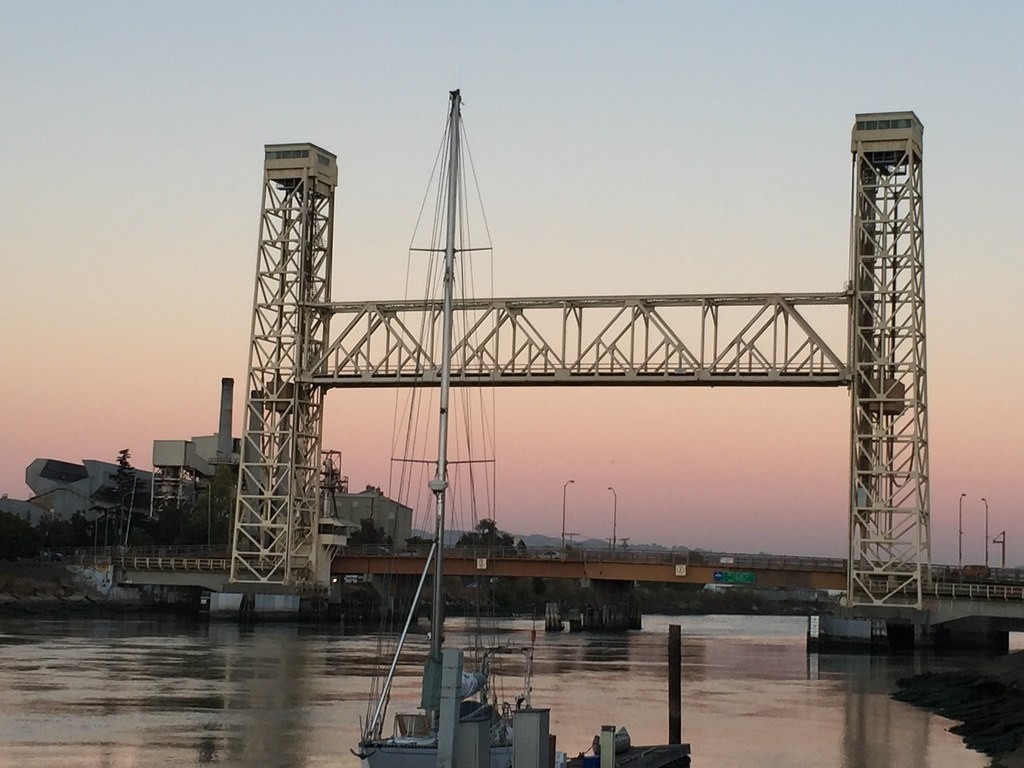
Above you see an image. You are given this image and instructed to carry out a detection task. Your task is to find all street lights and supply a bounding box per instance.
[959,493,965,565]
[608,486,616,549]
[981,498,989,566]
[561,479,574,552]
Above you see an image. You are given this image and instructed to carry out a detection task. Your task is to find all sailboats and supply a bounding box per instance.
[357,88,553,768]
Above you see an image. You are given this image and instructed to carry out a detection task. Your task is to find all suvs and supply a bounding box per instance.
[954,564,990,580]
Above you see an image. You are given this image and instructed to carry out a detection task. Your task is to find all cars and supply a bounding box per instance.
[33,551,64,566]
[354,545,392,555]
[533,549,561,561]
